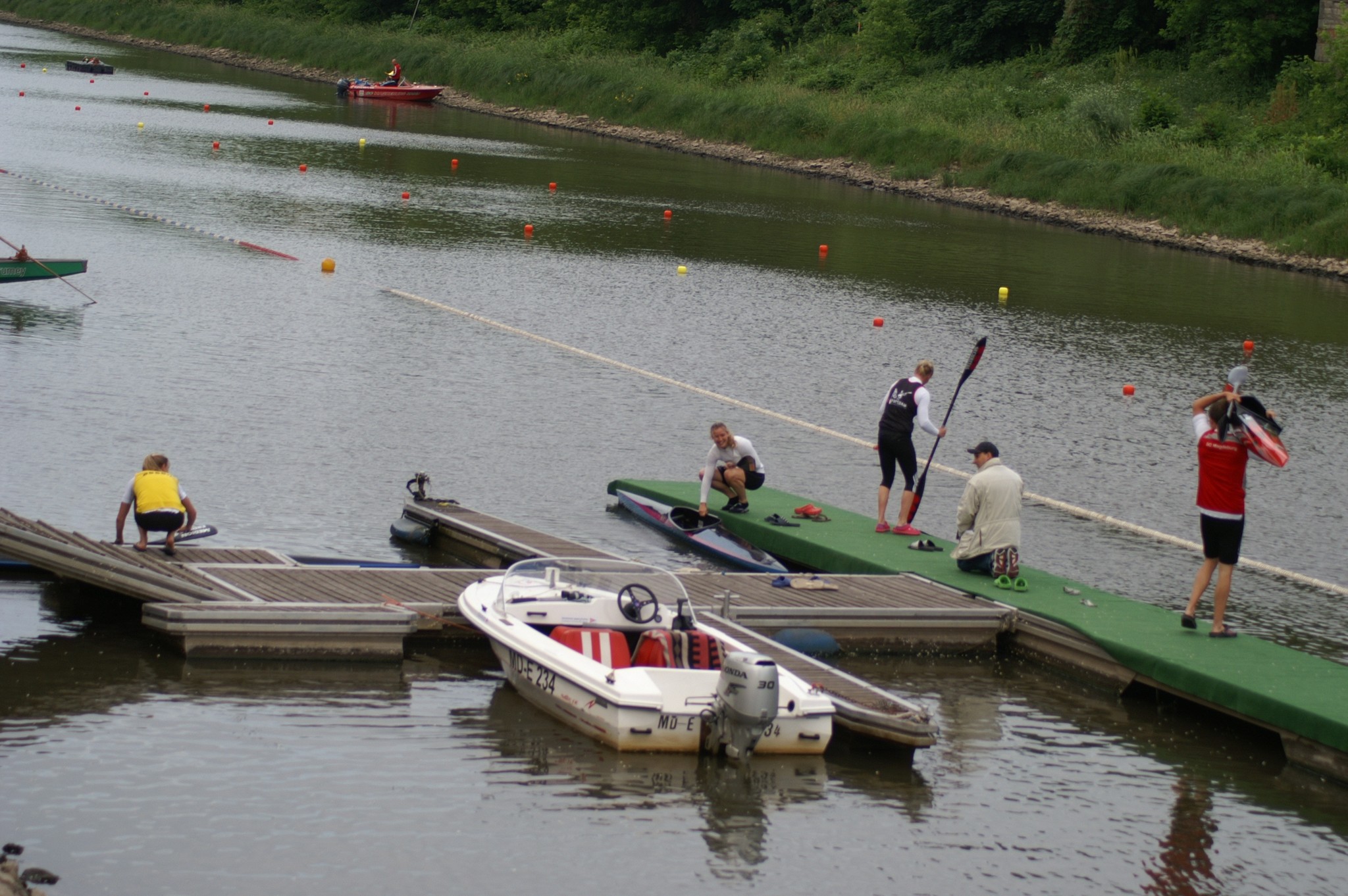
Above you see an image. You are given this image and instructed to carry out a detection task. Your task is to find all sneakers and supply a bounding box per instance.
[730,502,750,514]
[721,496,739,510]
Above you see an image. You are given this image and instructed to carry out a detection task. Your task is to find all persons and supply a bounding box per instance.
[1181,391,1275,637]
[389,58,401,86]
[82,56,105,66]
[699,422,766,518]
[873,361,948,536]
[113,453,197,556]
[950,441,1024,577]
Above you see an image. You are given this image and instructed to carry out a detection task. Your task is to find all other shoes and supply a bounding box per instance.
[1005,547,1019,578]
[991,548,1007,579]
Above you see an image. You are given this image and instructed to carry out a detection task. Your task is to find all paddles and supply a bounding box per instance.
[899,335,988,525]
[1218,364,1250,440]
[146,524,218,547]
[0,236,97,304]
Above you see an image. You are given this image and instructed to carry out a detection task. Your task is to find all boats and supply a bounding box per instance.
[698,611,945,749]
[337,78,446,102]
[65,59,114,75]
[613,487,797,575]
[0,257,102,288]
[455,554,839,767]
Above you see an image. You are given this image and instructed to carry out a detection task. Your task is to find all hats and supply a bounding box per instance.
[967,442,999,457]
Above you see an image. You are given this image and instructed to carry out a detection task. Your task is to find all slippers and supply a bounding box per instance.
[1209,623,1237,637]
[876,521,890,532]
[908,539,944,551]
[133,542,146,552]
[765,514,800,527]
[1014,577,1028,591]
[163,547,177,555]
[893,524,921,536]
[994,575,1011,589]
[1181,612,1197,629]
[791,504,831,522]
